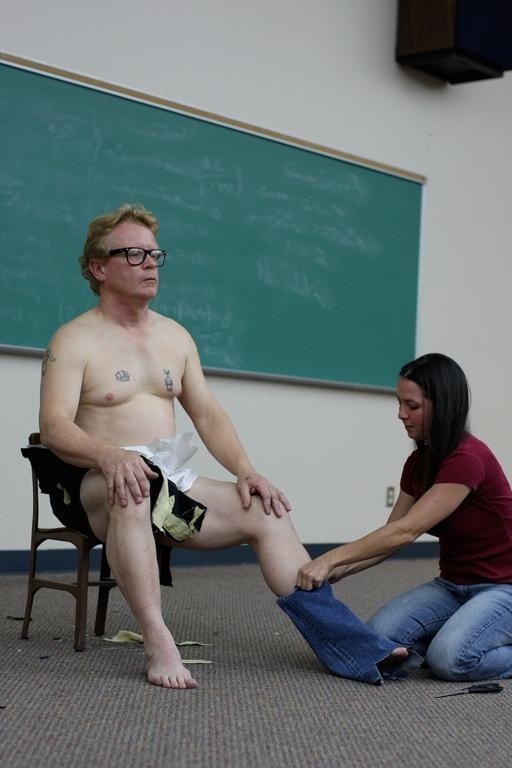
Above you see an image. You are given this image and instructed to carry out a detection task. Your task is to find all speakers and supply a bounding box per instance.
[395,0,512,85]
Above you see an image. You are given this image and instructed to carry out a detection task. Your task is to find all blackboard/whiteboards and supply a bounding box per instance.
[0,50,427,394]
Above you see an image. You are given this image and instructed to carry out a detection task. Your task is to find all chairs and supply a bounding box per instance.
[22,431,122,651]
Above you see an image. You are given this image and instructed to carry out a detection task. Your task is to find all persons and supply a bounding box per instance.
[38,202,412,691]
[294,352,511,683]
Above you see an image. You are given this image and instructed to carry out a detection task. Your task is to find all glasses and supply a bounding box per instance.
[109,246,167,268]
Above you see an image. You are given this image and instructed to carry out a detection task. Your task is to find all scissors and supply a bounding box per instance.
[434,684,504,697]
